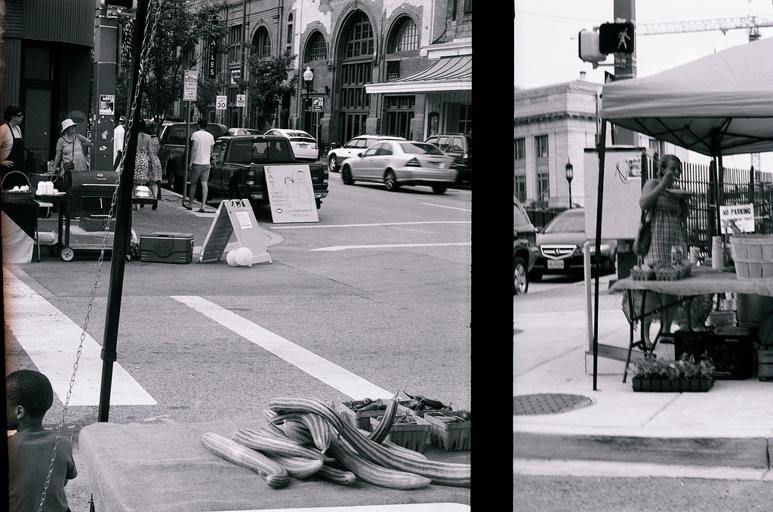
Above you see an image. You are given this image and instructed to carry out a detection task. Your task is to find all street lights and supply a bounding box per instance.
[564,157,574,208]
[302,65,314,131]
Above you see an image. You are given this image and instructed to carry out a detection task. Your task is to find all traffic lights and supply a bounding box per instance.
[577,29,608,64]
[600,21,635,55]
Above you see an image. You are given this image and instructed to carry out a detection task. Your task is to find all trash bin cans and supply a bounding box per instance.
[0,171,41,262]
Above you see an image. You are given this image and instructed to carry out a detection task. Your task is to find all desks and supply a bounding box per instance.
[611,267,773,384]
[30,173,60,218]
[0,200,53,262]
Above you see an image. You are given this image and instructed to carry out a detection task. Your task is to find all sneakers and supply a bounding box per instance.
[641,340,656,351]
[182,201,193,210]
[658,334,675,345]
[199,208,207,212]
[135,200,158,211]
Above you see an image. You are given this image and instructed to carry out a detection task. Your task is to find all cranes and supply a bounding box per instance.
[636,0,773,42]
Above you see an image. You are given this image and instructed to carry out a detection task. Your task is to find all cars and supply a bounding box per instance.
[529,209,620,280]
[340,140,459,196]
[114,116,329,212]
[686,203,717,245]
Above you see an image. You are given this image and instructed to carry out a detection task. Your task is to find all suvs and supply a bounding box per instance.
[423,132,472,188]
[327,135,406,172]
[513,195,538,297]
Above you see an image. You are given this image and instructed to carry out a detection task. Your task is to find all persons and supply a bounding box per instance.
[621,154,693,352]
[616,239,629,279]
[0,105,25,180]
[183,118,216,212]
[113,116,163,211]
[627,240,637,277]
[110,100,113,111]
[52,118,91,221]
[263,142,278,163]
[6,369,76,511]
[106,101,110,109]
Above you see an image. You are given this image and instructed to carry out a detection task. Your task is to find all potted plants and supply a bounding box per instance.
[630,352,716,393]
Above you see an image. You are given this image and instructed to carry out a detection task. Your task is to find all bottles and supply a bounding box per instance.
[37,181,55,196]
[670,245,683,266]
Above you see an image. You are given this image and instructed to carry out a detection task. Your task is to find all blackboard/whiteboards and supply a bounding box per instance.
[263,165,320,224]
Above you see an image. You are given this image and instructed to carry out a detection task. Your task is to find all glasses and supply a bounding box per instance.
[15,114,22,118]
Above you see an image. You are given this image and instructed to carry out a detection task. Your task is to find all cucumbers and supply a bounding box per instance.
[200,396,471,490]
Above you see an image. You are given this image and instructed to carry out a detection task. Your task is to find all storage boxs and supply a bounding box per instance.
[674,328,757,381]
[140,232,194,264]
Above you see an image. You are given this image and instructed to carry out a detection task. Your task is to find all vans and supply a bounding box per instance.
[725,198,769,228]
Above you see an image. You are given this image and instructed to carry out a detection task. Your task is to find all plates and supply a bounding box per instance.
[665,187,694,195]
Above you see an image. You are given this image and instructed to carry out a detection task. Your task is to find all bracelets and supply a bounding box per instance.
[660,181,669,187]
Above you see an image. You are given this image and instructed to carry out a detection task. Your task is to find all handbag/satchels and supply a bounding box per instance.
[63,160,74,171]
[632,220,653,258]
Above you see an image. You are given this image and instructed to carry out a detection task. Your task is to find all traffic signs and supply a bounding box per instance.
[215,95,227,110]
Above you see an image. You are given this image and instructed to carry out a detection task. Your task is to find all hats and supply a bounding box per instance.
[60,118,78,134]
[3,104,20,123]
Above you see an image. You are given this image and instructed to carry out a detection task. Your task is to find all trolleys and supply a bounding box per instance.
[60,172,135,263]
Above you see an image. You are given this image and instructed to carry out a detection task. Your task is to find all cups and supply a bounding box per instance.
[711,234,725,271]
[689,247,701,266]
